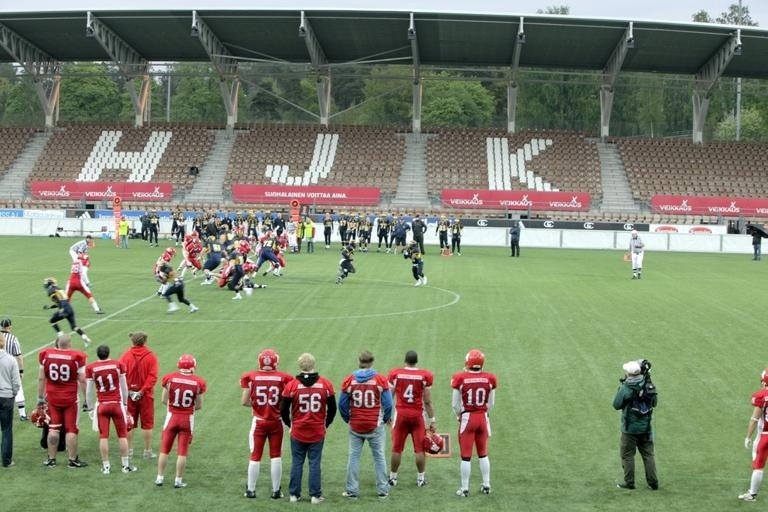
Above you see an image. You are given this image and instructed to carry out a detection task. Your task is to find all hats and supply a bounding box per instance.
[1,318,12,328]
[623,362,641,375]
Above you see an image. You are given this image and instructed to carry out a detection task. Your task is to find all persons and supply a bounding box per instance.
[273,212,286,235]
[66,251,101,314]
[148,210,160,247]
[510,223,521,257]
[140,211,150,240]
[0,333,20,467]
[280,353,336,505]
[198,235,226,285]
[450,218,462,256]
[363,216,373,246]
[630,230,646,279]
[69,236,93,267]
[84,345,138,475]
[117,216,130,249]
[175,213,186,246]
[254,231,275,268]
[285,215,299,253]
[1,316,29,423]
[411,216,428,254]
[336,241,358,285]
[151,246,176,299]
[224,245,246,301]
[170,211,178,238]
[221,214,233,229]
[338,216,348,250]
[160,264,198,312]
[263,216,273,231]
[304,216,316,253]
[347,217,357,245]
[234,212,243,236]
[752,226,763,261]
[176,239,202,273]
[36,335,90,467]
[294,217,304,253]
[385,350,435,488]
[193,212,221,235]
[216,253,257,288]
[322,212,334,248]
[404,239,426,288]
[451,351,496,496]
[394,217,410,254]
[177,231,200,279]
[264,234,288,277]
[358,220,367,251]
[39,333,66,452]
[251,235,283,277]
[116,330,155,458]
[153,353,206,489]
[737,367,768,503]
[246,211,259,240]
[40,276,90,346]
[389,215,398,253]
[238,350,294,500]
[376,214,390,252]
[338,351,394,498]
[436,214,450,254]
[614,358,661,491]
[745,219,752,237]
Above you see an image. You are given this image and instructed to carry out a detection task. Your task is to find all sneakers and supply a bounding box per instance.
[95,308,105,314]
[270,485,285,499]
[67,455,89,468]
[84,337,91,347]
[415,280,422,287]
[310,494,326,504]
[143,449,157,459]
[121,464,138,473]
[342,491,359,500]
[20,414,29,421]
[129,449,134,459]
[167,305,180,313]
[480,484,492,494]
[261,284,267,288]
[42,457,57,467]
[2,460,16,468]
[455,487,470,498]
[416,478,427,487]
[647,483,659,490]
[174,479,188,489]
[155,478,164,485]
[737,491,757,503]
[421,277,426,285]
[388,474,398,488]
[243,484,257,499]
[378,492,388,499]
[232,294,241,300]
[189,305,199,313]
[616,482,636,491]
[289,494,302,502]
[101,464,111,474]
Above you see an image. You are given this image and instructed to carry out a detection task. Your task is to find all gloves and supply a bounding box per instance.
[744,438,751,449]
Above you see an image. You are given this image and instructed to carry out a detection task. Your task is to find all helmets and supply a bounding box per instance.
[209,235,216,241]
[124,412,134,432]
[265,231,272,238]
[760,368,768,387]
[464,350,485,371]
[191,231,199,236]
[178,354,196,374]
[424,430,444,454]
[257,348,280,371]
[78,253,88,259]
[167,247,176,256]
[42,277,57,285]
[243,261,255,273]
[30,407,51,428]
[408,240,417,245]
[222,224,229,232]
